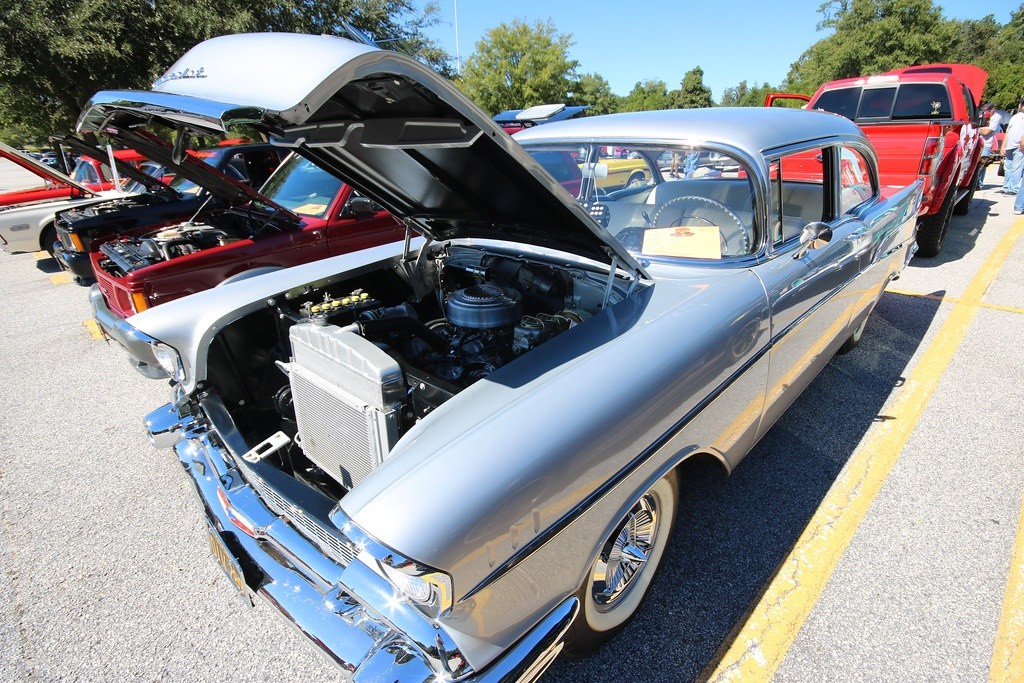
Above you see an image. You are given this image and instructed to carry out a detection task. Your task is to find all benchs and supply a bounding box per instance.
[645,180,823,224]
[650,181,836,224]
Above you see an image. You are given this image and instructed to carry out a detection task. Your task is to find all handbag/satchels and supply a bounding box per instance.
[998,162,1004,177]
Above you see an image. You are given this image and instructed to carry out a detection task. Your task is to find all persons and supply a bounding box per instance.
[929,124,976,194]
[976,98,1024,215]
[668,151,700,179]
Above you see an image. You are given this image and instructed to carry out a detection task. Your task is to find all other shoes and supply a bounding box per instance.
[674,175,682,180]
[994,190,1006,194]
[1014,209,1024,215]
[669,173,674,178]
[1004,192,1017,197]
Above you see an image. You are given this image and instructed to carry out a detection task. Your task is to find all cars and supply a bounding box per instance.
[0,101,740,321]
[71,31,928,682]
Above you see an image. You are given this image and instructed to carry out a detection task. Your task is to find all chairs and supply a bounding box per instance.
[680,200,805,257]
[597,201,686,256]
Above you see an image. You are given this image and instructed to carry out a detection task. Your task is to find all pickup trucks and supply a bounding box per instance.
[737,62,990,259]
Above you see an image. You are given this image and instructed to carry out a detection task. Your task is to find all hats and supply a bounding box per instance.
[981,103,994,112]
